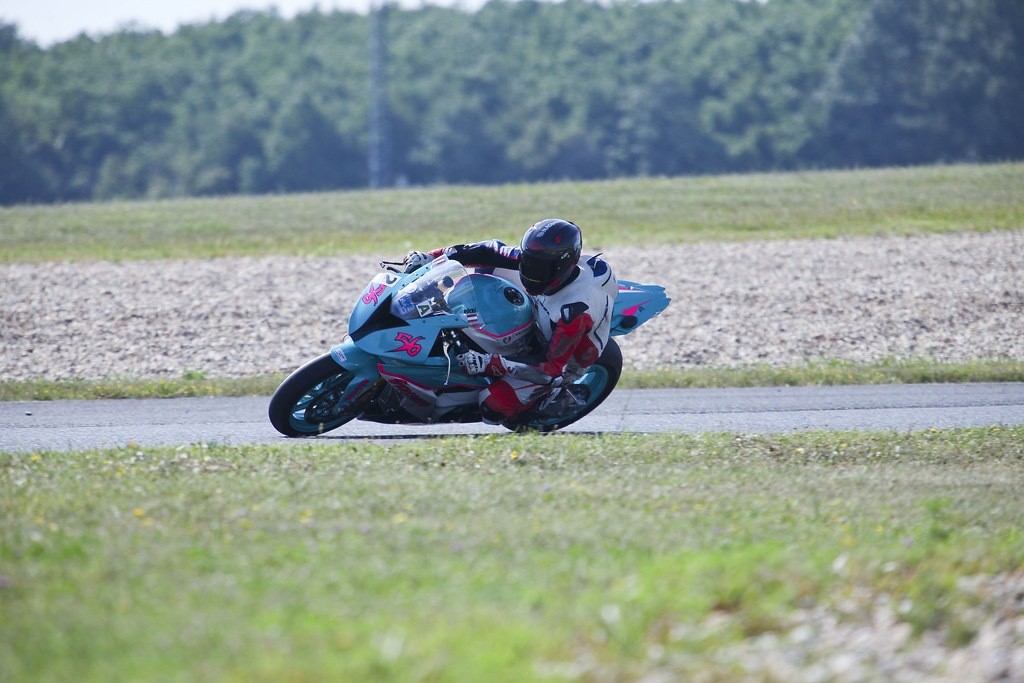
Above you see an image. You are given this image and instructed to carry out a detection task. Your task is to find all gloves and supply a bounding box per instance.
[401,246,457,274]
[456,348,507,375]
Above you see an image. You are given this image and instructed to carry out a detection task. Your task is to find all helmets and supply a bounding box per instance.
[518,218,582,296]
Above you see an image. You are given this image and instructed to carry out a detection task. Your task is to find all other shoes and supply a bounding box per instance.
[481,410,508,425]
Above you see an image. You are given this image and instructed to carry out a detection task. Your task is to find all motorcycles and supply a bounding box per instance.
[268,253,672,437]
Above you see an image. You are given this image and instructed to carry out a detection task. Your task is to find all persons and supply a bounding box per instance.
[402,218,619,426]
